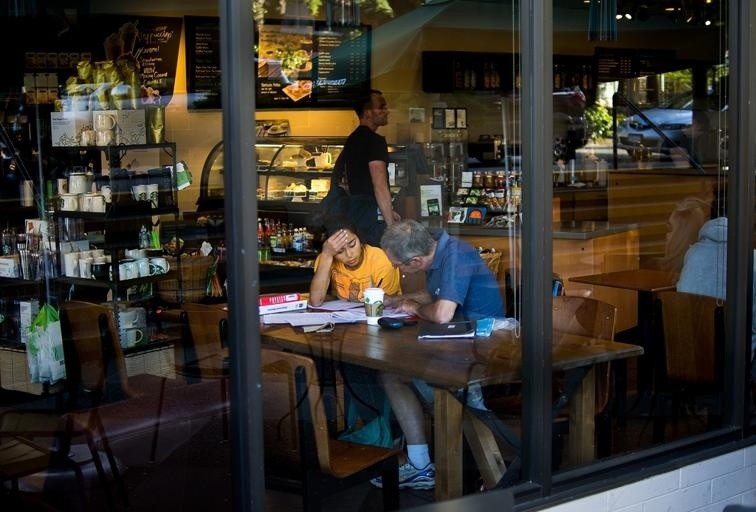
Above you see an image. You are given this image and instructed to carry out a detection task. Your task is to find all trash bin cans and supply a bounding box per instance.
[479,246,502,279]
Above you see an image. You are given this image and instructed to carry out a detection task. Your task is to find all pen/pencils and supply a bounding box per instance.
[376,278,383,288]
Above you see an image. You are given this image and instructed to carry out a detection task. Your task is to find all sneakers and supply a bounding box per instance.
[368,460,439,489]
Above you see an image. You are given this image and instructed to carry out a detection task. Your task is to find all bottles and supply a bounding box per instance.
[1,223,58,281]
[257,218,307,252]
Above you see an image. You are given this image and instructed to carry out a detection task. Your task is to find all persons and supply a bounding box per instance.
[660,110,709,164]
[676,216,728,300]
[335,90,401,247]
[310,222,401,425]
[370,219,504,490]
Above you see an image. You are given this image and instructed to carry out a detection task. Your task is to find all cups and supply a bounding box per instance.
[118,307,146,349]
[364,287,385,325]
[57,169,170,282]
[81,114,116,145]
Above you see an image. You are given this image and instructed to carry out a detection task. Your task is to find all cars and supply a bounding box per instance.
[495,87,590,166]
[617,86,715,160]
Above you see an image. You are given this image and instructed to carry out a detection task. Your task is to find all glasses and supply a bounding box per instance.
[392,255,418,270]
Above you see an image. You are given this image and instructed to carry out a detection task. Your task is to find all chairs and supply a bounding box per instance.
[220,343,407,510]
[659,290,724,381]
[552,292,616,431]
[53,296,192,395]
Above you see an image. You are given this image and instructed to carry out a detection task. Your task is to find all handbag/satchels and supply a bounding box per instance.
[306,183,356,238]
[339,415,395,449]
[25,304,68,386]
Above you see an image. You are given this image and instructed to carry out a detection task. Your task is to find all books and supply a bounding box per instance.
[259,293,307,315]
[417,320,475,339]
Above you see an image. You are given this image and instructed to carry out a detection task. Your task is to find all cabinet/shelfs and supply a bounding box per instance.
[2,72,191,354]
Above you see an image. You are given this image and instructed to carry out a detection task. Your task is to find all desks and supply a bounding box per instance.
[261,290,646,502]
[569,265,686,372]
